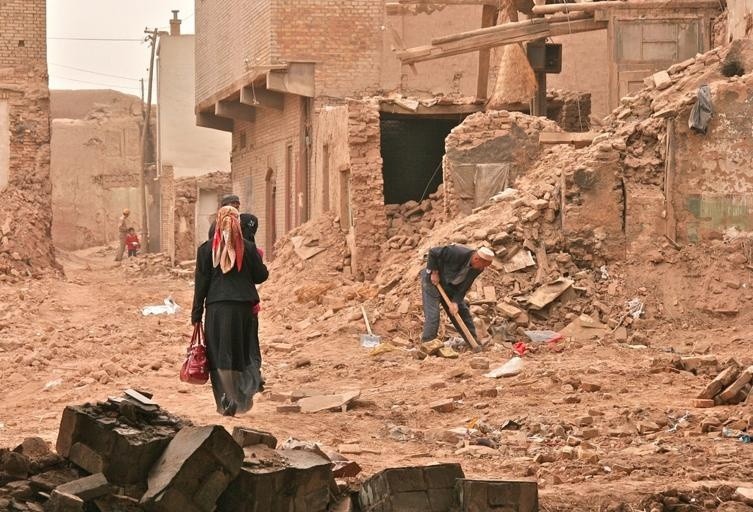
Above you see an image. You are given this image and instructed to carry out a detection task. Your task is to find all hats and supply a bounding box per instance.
[240,213,257,234]
[221,194,239,206]
[477,247,495,262]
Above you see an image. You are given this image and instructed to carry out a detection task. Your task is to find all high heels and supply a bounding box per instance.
[220,396,229,410]
[222,402,236,416]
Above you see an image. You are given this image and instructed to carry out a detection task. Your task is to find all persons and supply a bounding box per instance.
[209,196,240,240]
[115,208,132,261]
[191,206,268,416]
[241,214,265,393]
[125,229,142,254]
[420,245,495,342]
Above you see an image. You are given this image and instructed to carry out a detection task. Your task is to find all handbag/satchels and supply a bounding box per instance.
[180,342,210,385]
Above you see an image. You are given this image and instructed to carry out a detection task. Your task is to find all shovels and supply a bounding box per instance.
[360,306,380,348]
